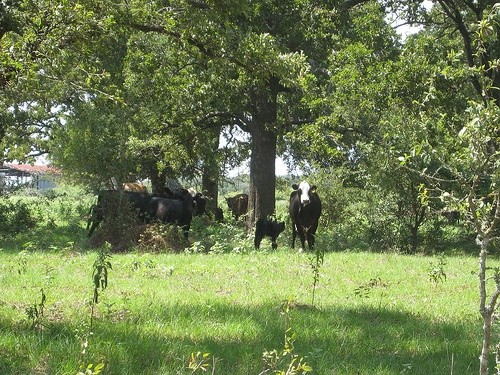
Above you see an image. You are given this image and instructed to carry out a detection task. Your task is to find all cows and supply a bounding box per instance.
[288,179,322,251]
[85,182,250,240]
[254,218,286,251]
